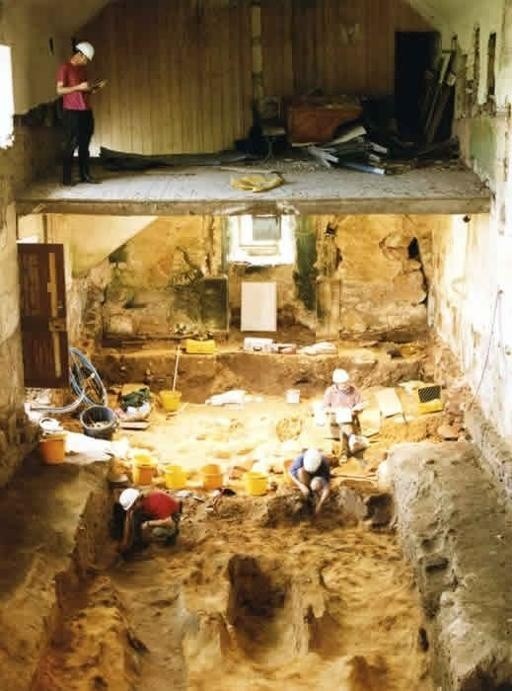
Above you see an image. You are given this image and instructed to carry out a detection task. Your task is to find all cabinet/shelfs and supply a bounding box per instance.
[284,105,362,141]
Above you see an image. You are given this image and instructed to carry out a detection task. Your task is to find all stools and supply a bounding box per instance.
[260,126,287,163]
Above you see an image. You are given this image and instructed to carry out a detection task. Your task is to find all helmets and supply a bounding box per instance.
[75,41,94,63]
[332,368,349,383]
[119,488,141,511]
[303,450,322,472]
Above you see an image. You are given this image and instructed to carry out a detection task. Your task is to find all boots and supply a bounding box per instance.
[78,150,97,183]
[62,151,73,185]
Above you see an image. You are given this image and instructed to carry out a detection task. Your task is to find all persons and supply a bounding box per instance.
[119,486,181,554]
[289,447,331,513]
[54,41,103,184]
[324,368,365,453]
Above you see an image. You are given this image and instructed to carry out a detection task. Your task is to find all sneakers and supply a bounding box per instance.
[166,530,179,545]
